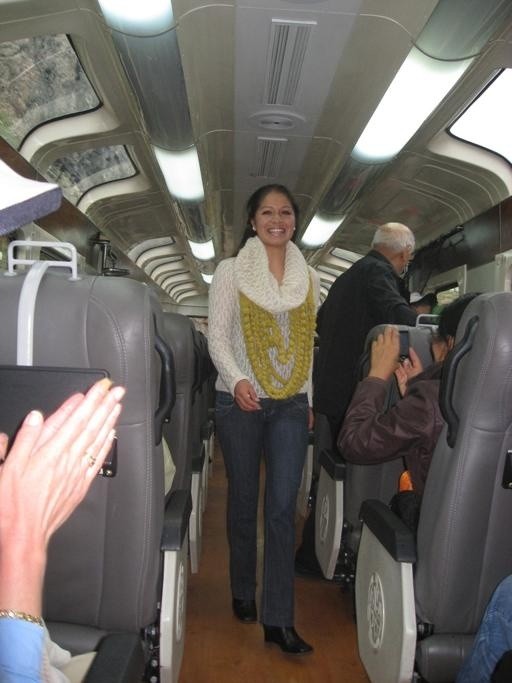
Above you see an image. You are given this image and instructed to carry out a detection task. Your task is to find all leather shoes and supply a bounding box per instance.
[264,625,314,656]
[231,596,258,624]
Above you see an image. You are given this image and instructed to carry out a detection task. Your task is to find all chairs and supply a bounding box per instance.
[0,241,161,681]
[315,315,439,585]
[356,291,510,683]
[188,331,214,573]
[154,310,194,682]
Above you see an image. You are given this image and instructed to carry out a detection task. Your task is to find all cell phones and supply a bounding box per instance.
[396,328,410,362]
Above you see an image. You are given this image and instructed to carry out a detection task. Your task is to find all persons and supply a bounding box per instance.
[455,574,512,683]
[208,185,320,655]
[409,290,439,314]
[337,290,483,525]
[0,375,128,677]
[294,222,432,578]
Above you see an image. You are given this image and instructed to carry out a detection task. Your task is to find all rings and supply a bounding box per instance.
[87,454,96,463]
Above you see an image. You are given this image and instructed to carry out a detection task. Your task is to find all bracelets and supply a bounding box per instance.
[0,608,44,626]
